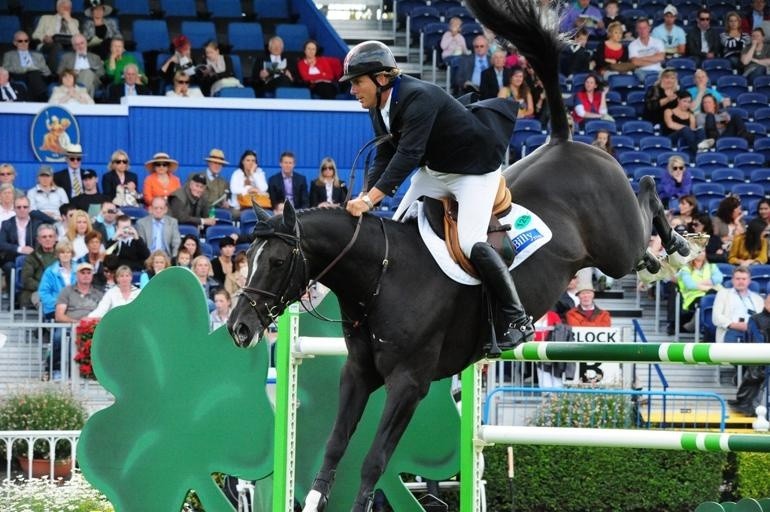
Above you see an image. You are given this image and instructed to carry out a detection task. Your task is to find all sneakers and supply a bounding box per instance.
[42,370,50,382]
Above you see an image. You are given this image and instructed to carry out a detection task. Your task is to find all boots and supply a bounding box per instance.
[470,240,536,351]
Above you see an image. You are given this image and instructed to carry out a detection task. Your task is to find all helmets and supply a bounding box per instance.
[337,40,398,83]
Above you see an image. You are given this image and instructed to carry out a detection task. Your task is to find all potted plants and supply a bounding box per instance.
[5,381,85,481]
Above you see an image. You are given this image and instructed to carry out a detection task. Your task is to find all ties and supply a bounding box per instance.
[129,86,134,95]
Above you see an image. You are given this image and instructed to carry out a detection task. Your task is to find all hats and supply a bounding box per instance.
[663,3,678,16]
[575,282,595,296]
[64,143,85,157]
[77,261,95,272]
[84,0,113,17]
[82,169,98,179]
[193,174,206,185]
[38,165,54,177]
[170,33,189,51]
[143,152,178,172]
[204,148,229,165]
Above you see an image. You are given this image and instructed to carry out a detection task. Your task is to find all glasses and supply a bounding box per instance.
[475,45,484,49]
[16,206,30,209]
[0,172,13,176]
[699,17,710,21]
[673,166,684,171]
[154,163,167,167]
[322,166,333,170]
[67,157,82,162]
[693,223,699,227]
[175,79,189,84]
[16,39,29,43]
[115,159,128,165]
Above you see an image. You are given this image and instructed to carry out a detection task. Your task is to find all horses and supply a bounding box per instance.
[227,1,710,512]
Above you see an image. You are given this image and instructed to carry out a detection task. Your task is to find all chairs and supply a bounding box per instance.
[611,74,636,91]
[276,24,311,53]
[717,75,748,103]
[638,136,672,162]
[725,279,758,300]
[741,214,762,226]
[158,53,179,79]
[89,17,119,45]
[124,50,147,79]
[15,257,36,315]
[207,0,247,19]
[644,73,658,92]
[251,0,292,21]
[753,137,770,160]
[619,152,651,181]
[16,0,56,14]
[628,91,648,117]
[161,0,197,19]
[691,183,725,211]
[686,168,709,185]
[656,152,690,184]
[723,107,748,132]
[461,23,485,56]
[695,154,728,179]
[678,137,698,161]
[748,199,769,213]
[743,122,766,146]
[688,10,719,28]
[3,16,24,47]
[573,135,592,147]
[573,74,594,94]
[210,207,238,227]
[701,294,727,343]
[670,199,702,213]
[445,6,476,33]
[715,263,737,282]
[582,118,617,140]
[749,263,770,281]
[724,12,750,31]
[177,225,197,251]
[218,86,255,101]
[737,93,767,119]
[749,169,769,193]
[44,82,87,104]
[132,18,171,54]
[755,107,770,130]
[407,6,443,71]
[528,135,551,155]
[241,209,277,235]
[429,1,462,20]
[710,168,745,192]
[117,203,148,234]
[512,120,541,148]
[607,106,635,126]
[621,9,647,27]
[654,10,684,28]
[636,167,667,188]
[715,137,749,165]
[733,184,767,211]
[702,59,730,83]
[226,21,265,52]
[681,75,712,93]
[667,59,694,70]
[220,53,244,83]
[607,136,636,161]
[421,22,449,88]
[114,0,151,16]
[752,75,769,98]
[272,85,314,102]
[393,0,421,47]
[182,20,216,53]
[620,121,655,144]
[709,199,724,215]
[206,226,243,254]
[734,153,766,179]
[608,92,623,107]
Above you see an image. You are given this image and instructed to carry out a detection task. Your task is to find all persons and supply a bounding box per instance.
[198,38,242,96]
[643,68,682,122]
[603,1,634,39]
[627,18,666,86]
[338,40,536,350]
[251,35,297,93]
[268,152,309,213]
[104,36,149,85]
[31,0,80,68]
[0,142,247,381]
[728,217,768,266]
[497,64,535,118]
[560,0,605,37]
[691,212,725,263]
[479,51,510,100]
[686,69,731,113]
[663,90,715,153]
[740,26,770,86]
[711,266,765,386]
[298,40,338,99]
[746,292,770,421]
[0,65,30,102]
[655,155,693,198]
[719,10,752,71]
[81,0,122,59]
[560,28,597,77]
[164,71,204,98]
[47,68,97,106]
[104,63,148,104]
[565,281,612,327]
[650,4,687,60]
[230,149,270,210]
[454,35,492,96]
[696,93,757,146]
[676,238,724,331]
[572,74,614,129]
[440,17,471,89]
[757,197,770,225]
[309,157,348,208]
[687,9,721,68]
[744,0,770,30]
[671,195,699,234]
[710,196,748,236]
[56,33,105,99]
[160,32,198,84]
[597,21,633,79]
[2,30,51,77]
